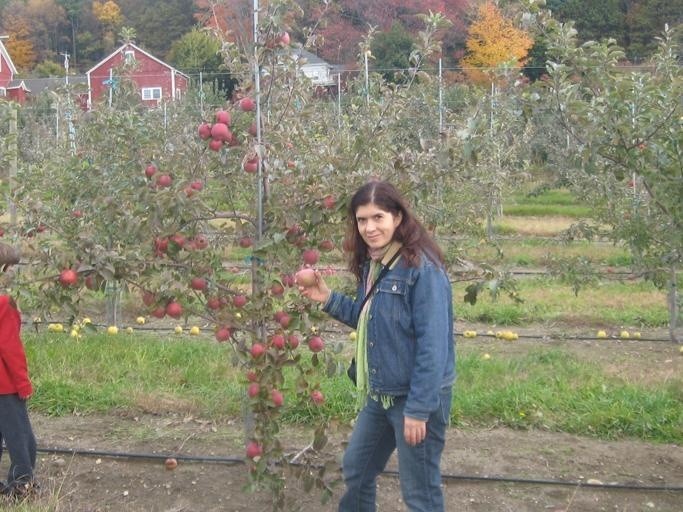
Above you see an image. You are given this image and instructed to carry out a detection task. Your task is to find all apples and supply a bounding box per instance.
[144,166,173,187]
[321,193,336,209]
[182,182,205,199]
[198,97,257,151]
[243,155,258,172]
[59,268,79,288]
[141,226,354,460]
[27,209,82,237]
[84,275,95,290]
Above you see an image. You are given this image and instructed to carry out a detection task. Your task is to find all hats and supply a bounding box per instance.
[0,242,20,265]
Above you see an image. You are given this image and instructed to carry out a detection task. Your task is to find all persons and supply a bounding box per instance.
[0,241,50,501]
[293,180,455,511]
